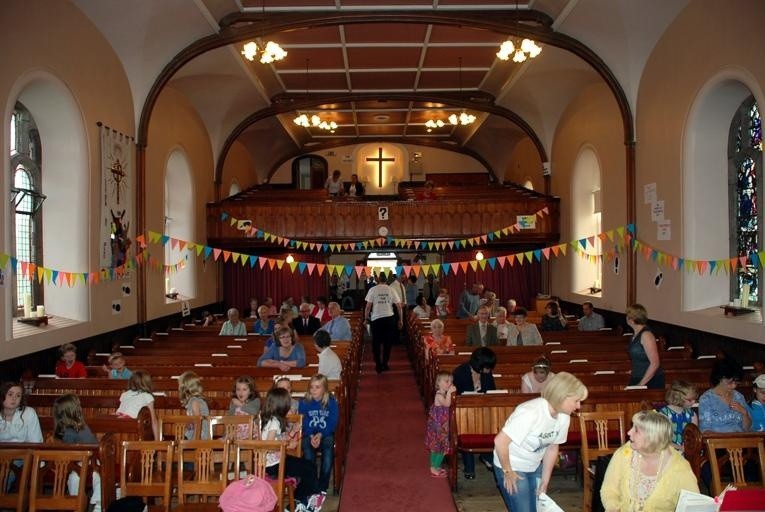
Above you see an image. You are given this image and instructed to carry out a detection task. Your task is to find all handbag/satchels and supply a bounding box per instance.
[219,477,278,511]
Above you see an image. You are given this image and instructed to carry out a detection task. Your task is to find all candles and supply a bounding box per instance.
[171,287,176,294]
[22,291,31,318]
[165,278,170,294]
[593,280,601,289]
[36,305,45,317]
[729,283,751,308]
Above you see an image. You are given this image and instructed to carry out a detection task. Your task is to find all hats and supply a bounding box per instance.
[752,374,765,388]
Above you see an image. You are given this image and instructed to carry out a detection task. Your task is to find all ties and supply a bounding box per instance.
[303,319,308,328]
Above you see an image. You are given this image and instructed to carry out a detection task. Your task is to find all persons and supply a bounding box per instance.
[1,325,342,512]
[110,208,132,267]
[406,272,765,512]
[324,169,343,200]
[206,270,419,373]
[345,174,365,201]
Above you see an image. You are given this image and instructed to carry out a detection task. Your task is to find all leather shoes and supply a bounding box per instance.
[464,456,494,479]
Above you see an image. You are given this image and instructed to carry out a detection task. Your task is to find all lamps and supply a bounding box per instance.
[293,58,340,131]
[475,249,485,260]
[424,56,477,129]
[241,5,288,64]
[495,1,543,64]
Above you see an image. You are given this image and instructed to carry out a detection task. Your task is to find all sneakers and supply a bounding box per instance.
[376,365,389,371]
[284,490,326,511]
[431,467,448,478]
